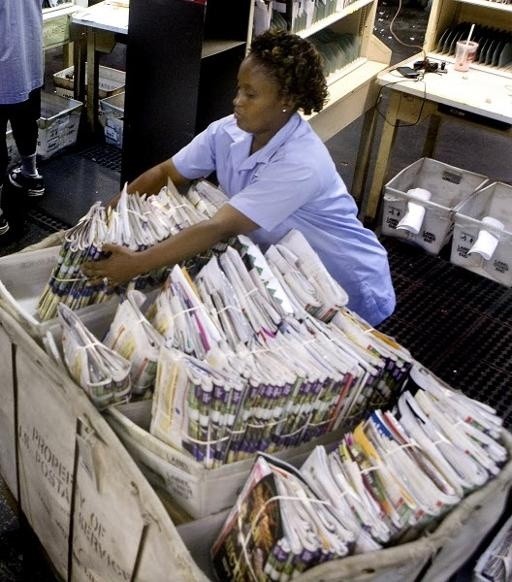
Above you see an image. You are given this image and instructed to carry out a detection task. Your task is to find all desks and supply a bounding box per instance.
[71,2,130,137]
[362,54,512,228]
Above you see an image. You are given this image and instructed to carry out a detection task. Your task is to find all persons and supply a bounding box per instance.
[0,0,48,238]
[81,27,400,331]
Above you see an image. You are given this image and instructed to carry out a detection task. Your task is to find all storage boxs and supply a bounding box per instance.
[6,60,127,172]
[381,155,512,290]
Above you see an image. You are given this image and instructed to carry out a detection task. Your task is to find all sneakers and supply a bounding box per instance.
[9,167,45,197]
[0,211,9,235]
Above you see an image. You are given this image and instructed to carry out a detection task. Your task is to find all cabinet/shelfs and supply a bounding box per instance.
[423,0,512,74]
[121,0,393,200]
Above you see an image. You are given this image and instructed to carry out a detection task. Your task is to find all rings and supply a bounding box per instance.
[103,277,111,288]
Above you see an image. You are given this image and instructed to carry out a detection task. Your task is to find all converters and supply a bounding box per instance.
[413,60,438,72]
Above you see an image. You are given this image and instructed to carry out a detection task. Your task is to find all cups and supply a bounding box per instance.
[454,40,480,73]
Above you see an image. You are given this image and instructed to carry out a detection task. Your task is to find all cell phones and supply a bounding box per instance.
[396,67,419,78]
